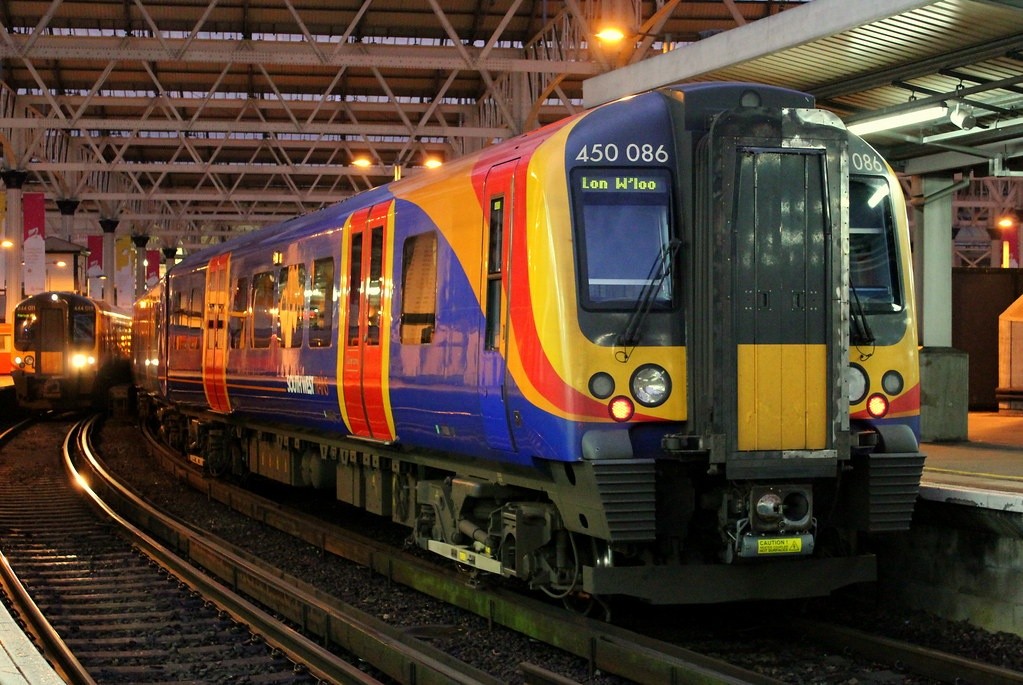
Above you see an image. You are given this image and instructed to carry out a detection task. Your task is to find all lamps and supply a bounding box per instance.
[86,275,108,280]
[53,260,66,266]
[594,28,660,43]
[400,159,442,169]
[0,241,14,248]
[846,101,950,137]
[999,218,1022,228]
[350,160,396,166]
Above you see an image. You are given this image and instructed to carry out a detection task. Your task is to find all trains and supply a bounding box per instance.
[132,81,927,623]
[10,290,133,416]
